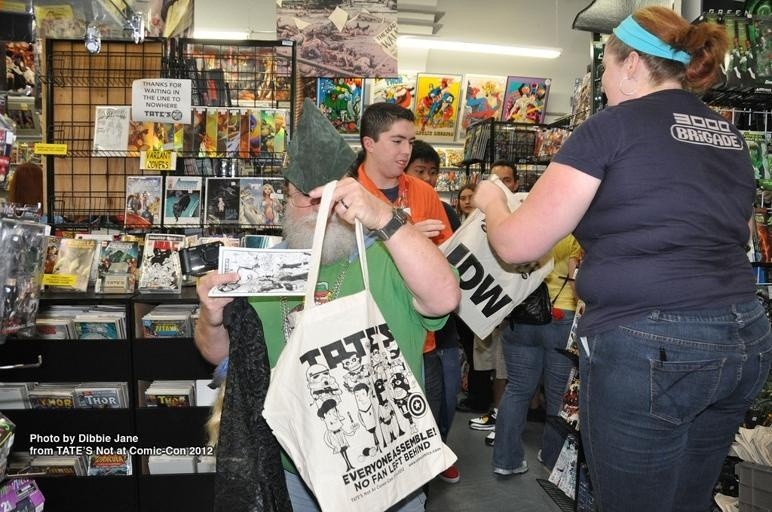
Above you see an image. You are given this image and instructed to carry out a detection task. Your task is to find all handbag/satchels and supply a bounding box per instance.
[438,174,555,340]
[262,287,459,512]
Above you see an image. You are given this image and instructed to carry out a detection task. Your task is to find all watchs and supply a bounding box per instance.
[376,207,408,241]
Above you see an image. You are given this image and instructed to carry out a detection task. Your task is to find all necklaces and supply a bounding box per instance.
[281,263,348,342]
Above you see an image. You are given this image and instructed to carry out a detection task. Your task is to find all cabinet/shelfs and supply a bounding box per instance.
[586,1,771,312]
[45,35,296,237]
[0,281,217,512]
[451,117,544,195]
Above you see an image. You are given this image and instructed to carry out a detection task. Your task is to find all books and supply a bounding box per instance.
[318,71,593,193]
[547,298,585,502]
[688,12,772,312]
[207,245,312,297]
[1,41,292,476]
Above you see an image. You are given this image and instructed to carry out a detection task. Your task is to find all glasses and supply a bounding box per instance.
[282,189,320,208]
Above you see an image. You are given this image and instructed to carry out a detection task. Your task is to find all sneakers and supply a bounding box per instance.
[438,465,461,483]
[455,398,529,475]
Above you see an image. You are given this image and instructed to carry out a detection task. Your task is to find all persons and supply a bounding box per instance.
[351,102,455,438]
[403,140,582,484]
[472,7,772,511]
[194,177,460,512]
[306,348,416,471]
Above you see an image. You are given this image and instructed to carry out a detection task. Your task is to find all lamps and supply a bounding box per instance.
[395,2,566,62]
